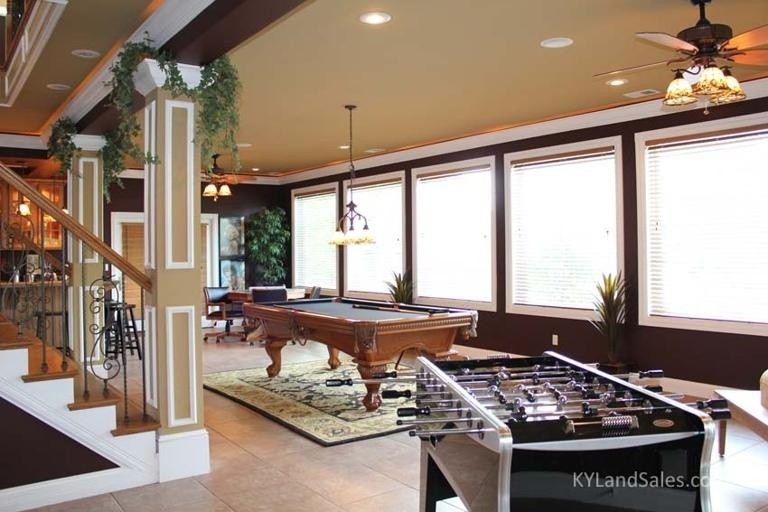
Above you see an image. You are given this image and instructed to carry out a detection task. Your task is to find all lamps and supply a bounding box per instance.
[15,201,32,216]
[48,206,69,221]
[202,152,232,202]
[328,104,376,245]
[663,60,747,114]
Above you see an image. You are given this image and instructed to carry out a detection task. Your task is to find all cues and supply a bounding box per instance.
[352,305,429,314]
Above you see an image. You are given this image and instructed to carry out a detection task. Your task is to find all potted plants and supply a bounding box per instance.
[588,271,632,375]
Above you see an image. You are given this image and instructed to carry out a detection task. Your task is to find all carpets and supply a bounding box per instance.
[203,355,421,446]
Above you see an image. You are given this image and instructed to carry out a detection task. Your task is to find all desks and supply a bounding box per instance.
[713,390,767,458]
[242,296,479,411]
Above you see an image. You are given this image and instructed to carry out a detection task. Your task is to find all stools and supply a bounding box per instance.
[34,310,69,354]
[105,300,142,359]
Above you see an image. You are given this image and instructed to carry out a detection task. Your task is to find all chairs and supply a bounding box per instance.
[203,287,321,346]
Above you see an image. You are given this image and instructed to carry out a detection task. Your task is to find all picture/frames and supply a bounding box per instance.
[219,216,247,258]
[220,260,247,290]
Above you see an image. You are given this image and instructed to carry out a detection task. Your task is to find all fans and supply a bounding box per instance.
[591,1,768,77]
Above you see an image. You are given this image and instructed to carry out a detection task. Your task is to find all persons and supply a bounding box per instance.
[219,222,244,257]
[221,264,245,291]
